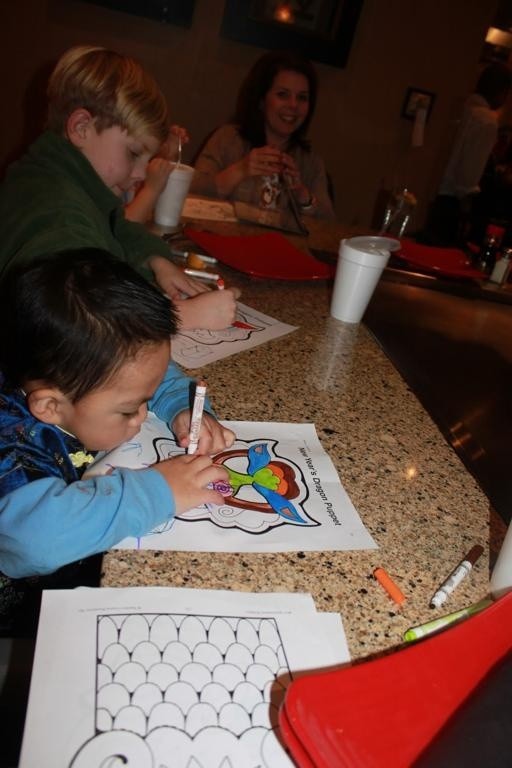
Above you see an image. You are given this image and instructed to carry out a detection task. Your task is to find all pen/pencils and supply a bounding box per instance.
[403,599,493,643]
[187,380,207,455]
[430,544,484,609]
[171,250,225,290]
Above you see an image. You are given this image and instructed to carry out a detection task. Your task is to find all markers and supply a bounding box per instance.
[371,565,406,604]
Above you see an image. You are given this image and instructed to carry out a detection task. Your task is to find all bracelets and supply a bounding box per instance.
[302,193,317,211]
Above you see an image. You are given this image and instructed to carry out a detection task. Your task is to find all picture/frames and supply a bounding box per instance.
[217,0,363,68]
[401,85,436,125]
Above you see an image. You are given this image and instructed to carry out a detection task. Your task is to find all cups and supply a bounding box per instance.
[151,160,196,227]
[375,186,414,244]
[330,237,389,326]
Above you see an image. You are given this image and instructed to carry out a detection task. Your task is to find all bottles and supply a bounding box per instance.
[472,223,511,285]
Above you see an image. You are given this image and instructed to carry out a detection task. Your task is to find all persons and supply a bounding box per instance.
[0,44,241,332]
[191,51,338,220]
[119,122,193,223]
[0,248,238,578]
[387,65,512,284]
